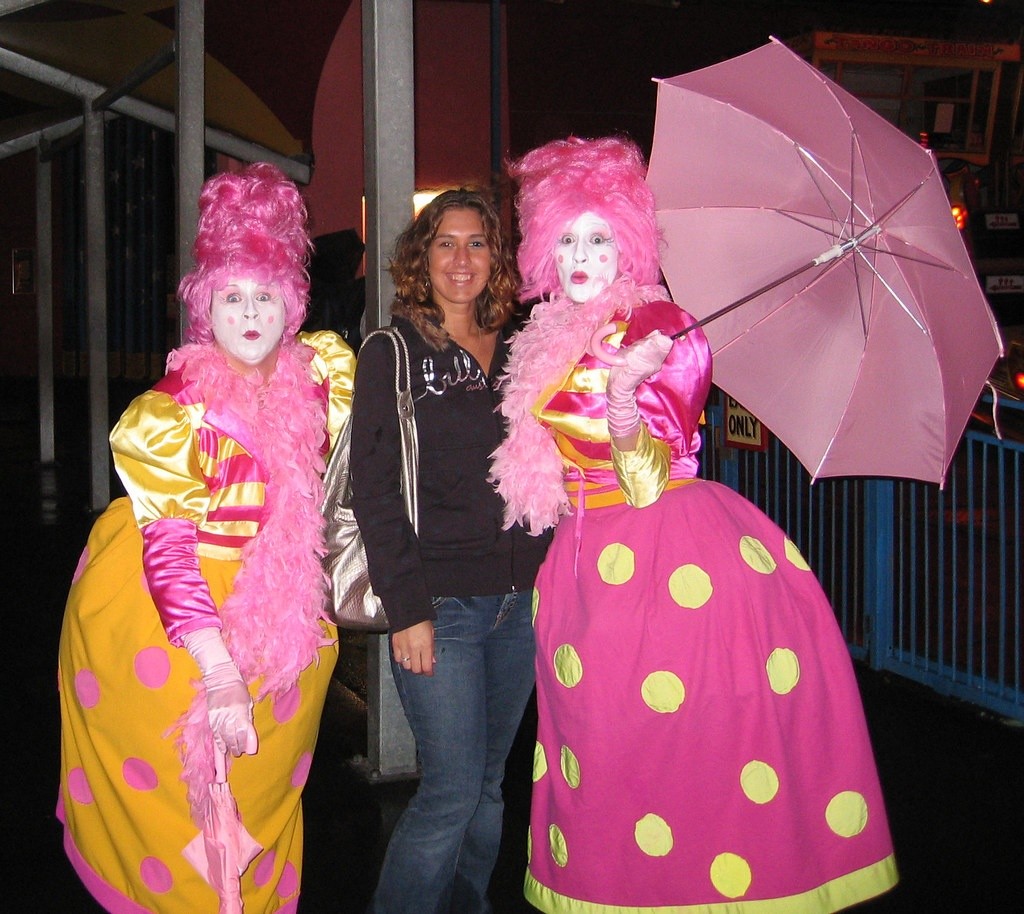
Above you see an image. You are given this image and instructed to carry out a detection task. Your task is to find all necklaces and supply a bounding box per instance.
[474,323,482,356]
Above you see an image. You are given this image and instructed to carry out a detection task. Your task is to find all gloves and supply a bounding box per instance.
[183,629,257,758]
[604,327,675,438]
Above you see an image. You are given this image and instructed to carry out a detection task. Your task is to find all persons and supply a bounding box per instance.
[351,188,552,914]
[57,163,360,914]
[488,134,898,914]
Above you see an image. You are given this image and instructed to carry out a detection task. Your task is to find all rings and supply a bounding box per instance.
[402,657,410,661]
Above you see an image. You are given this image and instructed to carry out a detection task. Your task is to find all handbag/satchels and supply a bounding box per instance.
[316,325,423,630]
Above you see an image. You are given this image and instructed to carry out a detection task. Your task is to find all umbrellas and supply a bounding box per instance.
[589,36,1004,490]
[184,721,265,914]
